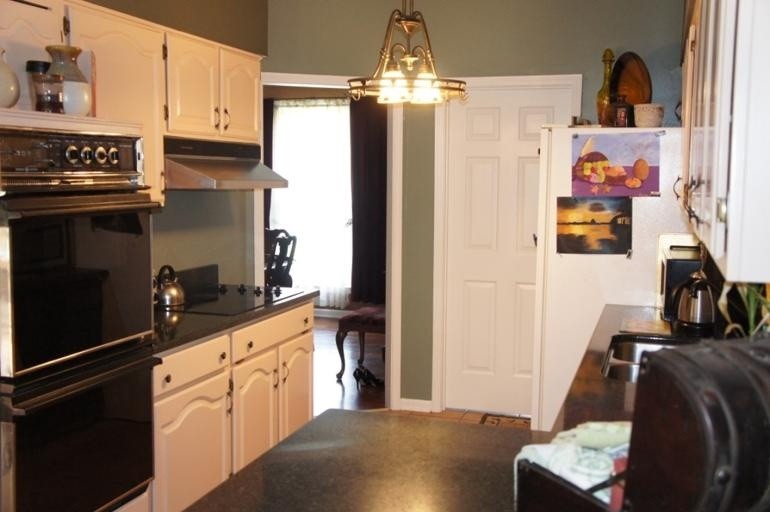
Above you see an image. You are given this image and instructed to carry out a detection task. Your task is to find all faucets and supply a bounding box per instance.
[721,324,748,336]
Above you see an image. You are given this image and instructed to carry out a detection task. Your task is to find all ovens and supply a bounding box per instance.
[1,120,154,511]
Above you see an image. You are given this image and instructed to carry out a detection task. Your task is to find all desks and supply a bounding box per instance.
[549,304,671,442]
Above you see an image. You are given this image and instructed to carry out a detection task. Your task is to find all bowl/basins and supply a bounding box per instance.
[634,104,664,127]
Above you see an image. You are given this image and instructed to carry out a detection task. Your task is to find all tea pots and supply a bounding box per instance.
[158,305,183,341]
[154,265,185,309]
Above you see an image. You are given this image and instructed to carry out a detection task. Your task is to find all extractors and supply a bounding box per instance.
[162,136,291,191]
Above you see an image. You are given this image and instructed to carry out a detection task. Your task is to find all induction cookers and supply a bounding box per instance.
[180,281,304,318]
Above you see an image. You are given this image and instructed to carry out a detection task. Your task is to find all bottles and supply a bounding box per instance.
[34,74,64,115]
[597,49,615,127]
[614,95,632,127]
[24,58,55,107]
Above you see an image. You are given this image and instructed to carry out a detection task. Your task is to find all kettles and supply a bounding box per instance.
[674,271,716,332]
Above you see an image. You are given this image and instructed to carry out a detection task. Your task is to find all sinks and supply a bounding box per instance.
[602,333,700,382]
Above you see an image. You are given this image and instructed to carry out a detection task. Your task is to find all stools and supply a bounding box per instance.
[334,307,385,379]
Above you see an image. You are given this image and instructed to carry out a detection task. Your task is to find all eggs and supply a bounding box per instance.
[633,158,649,181]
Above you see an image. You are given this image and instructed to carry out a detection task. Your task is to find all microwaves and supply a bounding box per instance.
[657,230,703,323]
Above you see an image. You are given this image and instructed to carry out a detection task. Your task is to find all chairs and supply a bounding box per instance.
[265,228,296,287]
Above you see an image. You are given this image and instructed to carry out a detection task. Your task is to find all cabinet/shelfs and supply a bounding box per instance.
[69,0,166,207]
[675,0,770,283]
[165,29,262,144]
[231,303,314,473]
[0,0,65,111]
[150,334,231,512]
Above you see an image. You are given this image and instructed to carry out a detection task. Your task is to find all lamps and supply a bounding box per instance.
[348,1,469,105]
